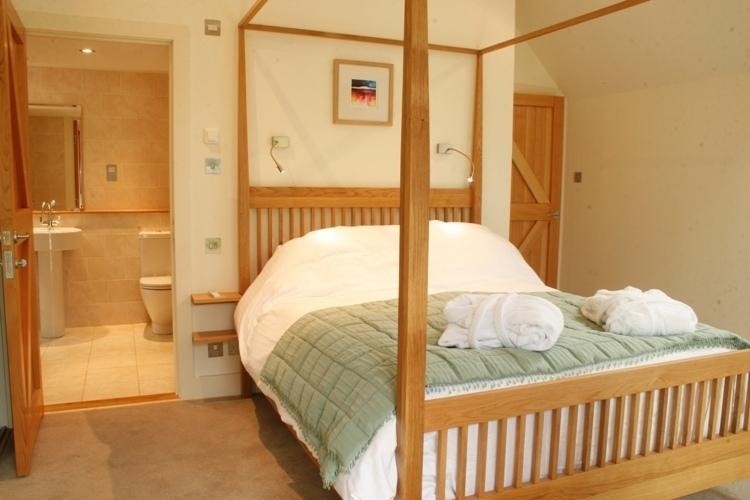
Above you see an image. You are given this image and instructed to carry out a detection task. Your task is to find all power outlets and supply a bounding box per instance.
[227,340,239,356]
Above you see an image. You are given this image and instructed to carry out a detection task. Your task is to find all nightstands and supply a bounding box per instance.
[189,290,244,345]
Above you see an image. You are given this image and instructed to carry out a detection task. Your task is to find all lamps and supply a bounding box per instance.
[437,142,478,182]
[268,135,289,175]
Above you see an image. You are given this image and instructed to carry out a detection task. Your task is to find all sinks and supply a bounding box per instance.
[34,227,82,252]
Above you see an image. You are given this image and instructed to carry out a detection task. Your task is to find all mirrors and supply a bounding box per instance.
[29,102,84,216]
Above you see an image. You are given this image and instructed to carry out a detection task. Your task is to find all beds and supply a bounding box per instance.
[232,0,749,500]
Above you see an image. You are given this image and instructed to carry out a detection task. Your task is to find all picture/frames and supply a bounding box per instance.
[331,58,395,128]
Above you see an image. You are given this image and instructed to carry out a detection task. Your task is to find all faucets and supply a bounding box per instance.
[39,202,52,229]
[51,199,56,212]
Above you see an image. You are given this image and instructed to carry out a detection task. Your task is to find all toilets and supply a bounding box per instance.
[139,231,173,336]
